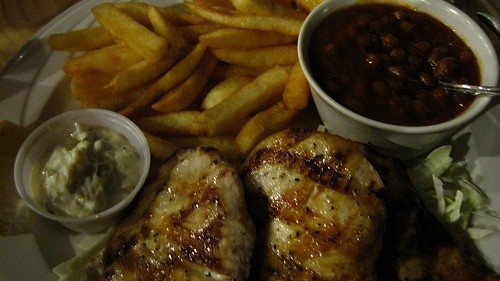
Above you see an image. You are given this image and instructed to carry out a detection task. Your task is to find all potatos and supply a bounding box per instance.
[45,0,327,161]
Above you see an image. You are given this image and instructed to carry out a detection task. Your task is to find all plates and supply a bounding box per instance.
[1,0,499,280]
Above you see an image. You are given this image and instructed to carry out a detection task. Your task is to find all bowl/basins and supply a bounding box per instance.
[15,109,150,233]
[297,1,499,161]
